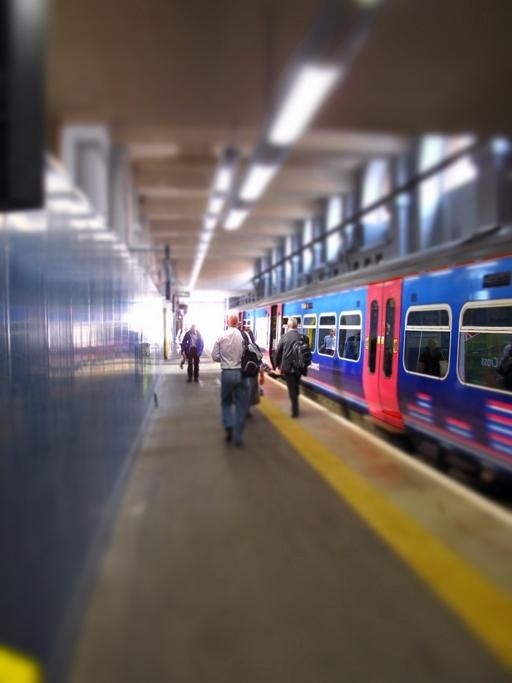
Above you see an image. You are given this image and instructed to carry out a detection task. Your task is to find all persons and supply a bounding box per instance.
[417,337,442,376]
[243,328,265,420]
[344,330,361,360]
[175,323,188,368]
[182,322,205,384]
[320,328,335,354]
[272,317,311,419]
[211,312,260,450]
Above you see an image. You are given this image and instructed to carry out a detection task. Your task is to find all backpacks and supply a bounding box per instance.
[283,331,313,371]
[238,328,260,378]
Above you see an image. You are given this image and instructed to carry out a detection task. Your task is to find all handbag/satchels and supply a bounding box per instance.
[244,330,263,362]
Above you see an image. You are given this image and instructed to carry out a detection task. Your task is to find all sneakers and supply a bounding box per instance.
[225,426,244,446]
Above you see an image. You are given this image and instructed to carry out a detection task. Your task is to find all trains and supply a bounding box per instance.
[223,223,512,476]
